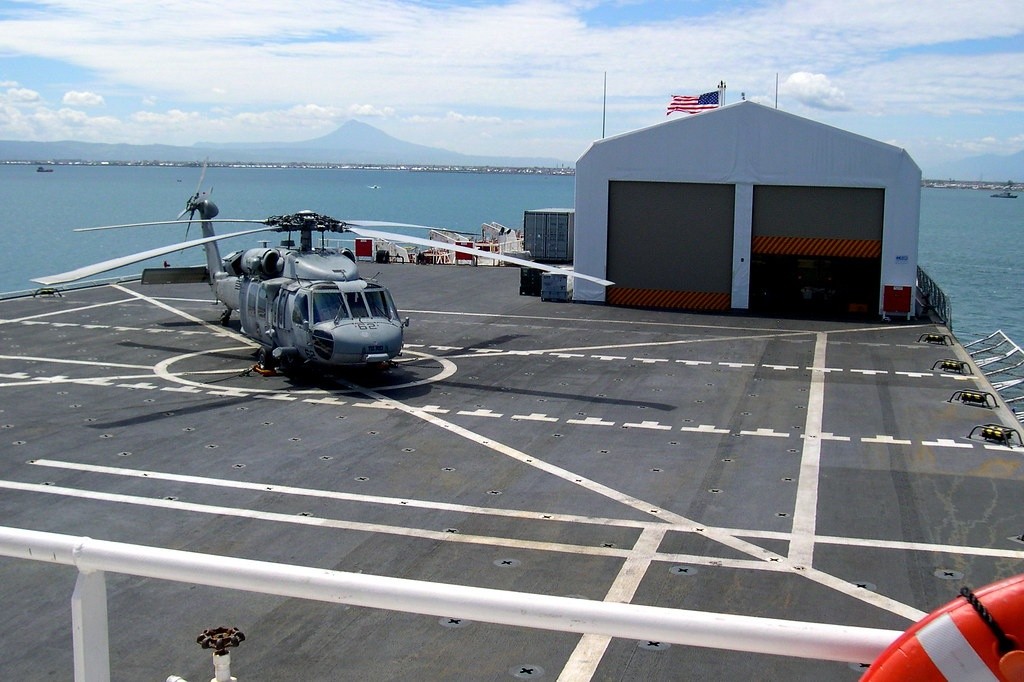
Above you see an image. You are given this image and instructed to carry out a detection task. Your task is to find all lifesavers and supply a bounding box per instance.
[860,573,1024,682]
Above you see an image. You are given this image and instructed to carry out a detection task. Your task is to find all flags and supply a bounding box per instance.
[666,89,718,114]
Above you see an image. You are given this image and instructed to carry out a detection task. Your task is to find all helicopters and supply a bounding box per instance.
[28,152,617,376]
